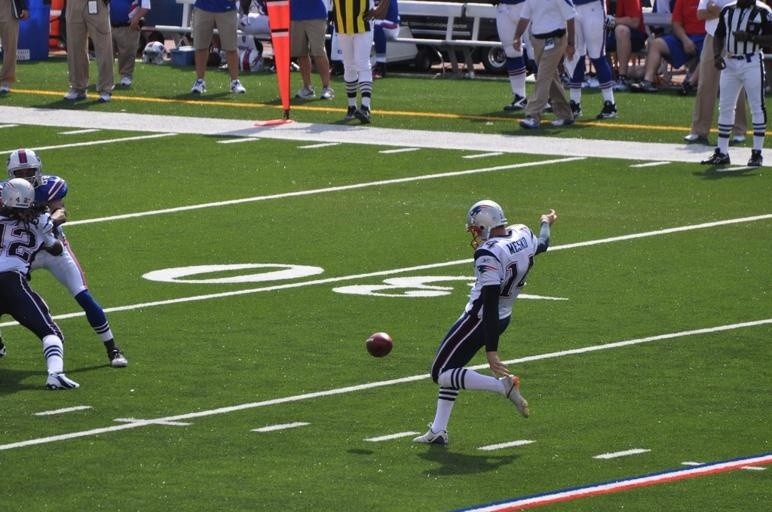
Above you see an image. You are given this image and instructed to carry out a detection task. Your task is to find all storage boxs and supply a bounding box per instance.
[171,44,218,66]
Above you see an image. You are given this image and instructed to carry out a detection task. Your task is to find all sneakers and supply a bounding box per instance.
[700,148,731,166]
[733,135,746,143]
[596,100,618,120]
[372,61,386,79]
[95,82,115,103]
[747,149,763,167]
[0,83,10,93]
[412,427,449,445]
[44,372,80,391]
[120,74,132,87]
[64,88,88,101]
[684,133,707,142]
[106,346,128,368]
[192,78,207,93]
[320,87,335,100]
[344,104,371,124]
[500,374,530,418]
[559,70,696,96]
[230,79,246,95]
[295,86,316,100]
[502,93,583,129]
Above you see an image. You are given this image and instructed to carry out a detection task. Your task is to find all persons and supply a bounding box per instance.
[0,147,129,369]
[681,0,747,144]
[412,198,557,446]
[0,176,79,388]
[697,0,772,168]
[491,0,648,129]
[630,0,708,95]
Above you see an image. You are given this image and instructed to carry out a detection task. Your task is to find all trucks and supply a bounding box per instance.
[387,0,514,80]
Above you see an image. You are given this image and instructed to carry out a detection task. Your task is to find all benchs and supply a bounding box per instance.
[447,2,772,79]
[153,0,466,78]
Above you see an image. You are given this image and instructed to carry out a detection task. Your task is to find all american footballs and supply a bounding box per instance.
[366,332,392,357]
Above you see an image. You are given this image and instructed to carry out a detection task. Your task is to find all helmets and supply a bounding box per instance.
[240,48,263,73]
[0,177,35,209]
[464,199,508,251]
[143,41,167,65]
[6,148,43,187]
